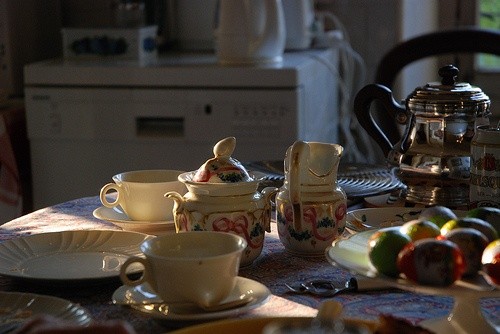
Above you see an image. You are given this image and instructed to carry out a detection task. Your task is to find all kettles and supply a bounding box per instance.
[353,65,491,206]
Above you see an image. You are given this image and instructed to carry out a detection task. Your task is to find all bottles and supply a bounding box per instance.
[285,0,313,51]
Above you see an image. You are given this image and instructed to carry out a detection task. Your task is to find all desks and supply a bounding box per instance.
[0,193,500,334]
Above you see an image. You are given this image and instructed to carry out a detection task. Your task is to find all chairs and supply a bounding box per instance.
[374,25,500,87]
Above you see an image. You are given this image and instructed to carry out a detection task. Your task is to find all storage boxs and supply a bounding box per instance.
[63,26,158,66]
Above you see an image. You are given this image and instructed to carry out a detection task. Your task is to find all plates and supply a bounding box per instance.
[110,276,272,329]
[0,231,159,285]
[168,318,374,334]
[345,209,468,235]
[93,205,175,231]
[0,291,93,334]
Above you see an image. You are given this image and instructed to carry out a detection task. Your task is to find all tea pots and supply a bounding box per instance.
[164,137,280,270]
[276,141,348,257]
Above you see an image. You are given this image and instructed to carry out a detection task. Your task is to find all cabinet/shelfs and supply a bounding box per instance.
[24,51,338,210]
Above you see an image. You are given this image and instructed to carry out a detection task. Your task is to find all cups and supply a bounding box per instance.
[99,169,188,221]
[120,231,248,311]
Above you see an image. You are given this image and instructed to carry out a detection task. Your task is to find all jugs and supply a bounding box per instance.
[214,0,287,65]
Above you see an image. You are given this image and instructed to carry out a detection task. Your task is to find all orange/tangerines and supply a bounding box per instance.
[366,205,500,275]
